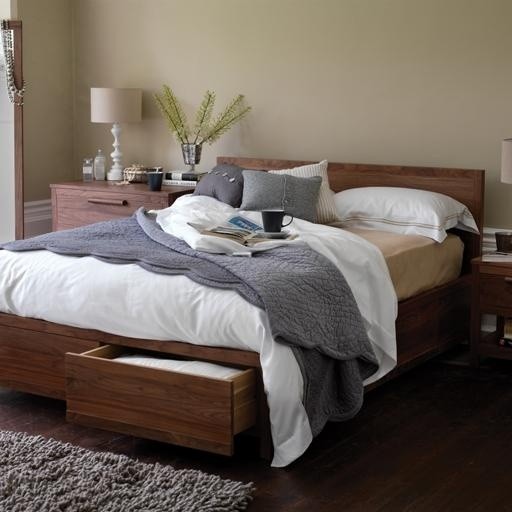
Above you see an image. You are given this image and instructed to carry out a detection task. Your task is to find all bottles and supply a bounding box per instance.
[93,148,106,182]
[83,159,93,181]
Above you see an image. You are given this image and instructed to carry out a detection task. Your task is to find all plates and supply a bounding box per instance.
[256,230,289,239]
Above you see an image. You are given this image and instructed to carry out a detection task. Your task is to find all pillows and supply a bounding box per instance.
[333,186,479,243]
[240,168,322,224]
[267,160,345,225]
[194,162,268,209]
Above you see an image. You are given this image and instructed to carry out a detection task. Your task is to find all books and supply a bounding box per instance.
[482,250,512,263]
[159,171,209,186]
[200,215,300,249]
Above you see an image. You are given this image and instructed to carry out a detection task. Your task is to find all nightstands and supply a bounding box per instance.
[49,180,195,232]
[470,250,512,371]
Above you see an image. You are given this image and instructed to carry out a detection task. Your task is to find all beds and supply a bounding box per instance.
[0,157,486,458]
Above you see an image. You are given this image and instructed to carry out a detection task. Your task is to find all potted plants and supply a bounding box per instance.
[154,84,252,173]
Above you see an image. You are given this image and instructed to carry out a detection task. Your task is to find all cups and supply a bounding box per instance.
[145,167,163,173]
[495,231,510,251]
[261,210,294,233]
[148,172,164,191]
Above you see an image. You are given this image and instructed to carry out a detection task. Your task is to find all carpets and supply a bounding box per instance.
[1,431,256,512]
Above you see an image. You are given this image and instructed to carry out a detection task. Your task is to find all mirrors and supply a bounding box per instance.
[0,19,23,247]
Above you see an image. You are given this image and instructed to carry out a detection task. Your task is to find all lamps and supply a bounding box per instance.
[500,137,512,185]
[90,87,143,181]
